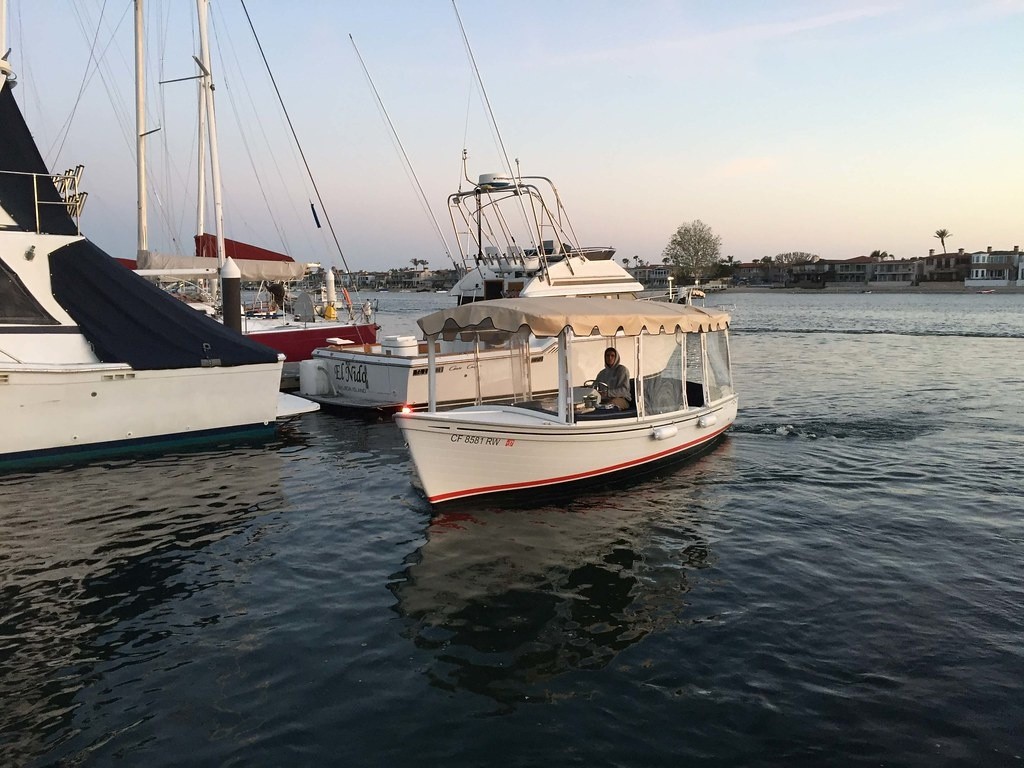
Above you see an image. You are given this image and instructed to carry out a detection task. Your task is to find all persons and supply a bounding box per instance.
[592,346,632,411]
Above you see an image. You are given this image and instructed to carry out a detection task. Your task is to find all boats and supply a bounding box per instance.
[293,158,693,414]
[390,296,740,504]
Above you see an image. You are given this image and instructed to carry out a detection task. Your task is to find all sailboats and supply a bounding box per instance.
[0,0,380,472]
